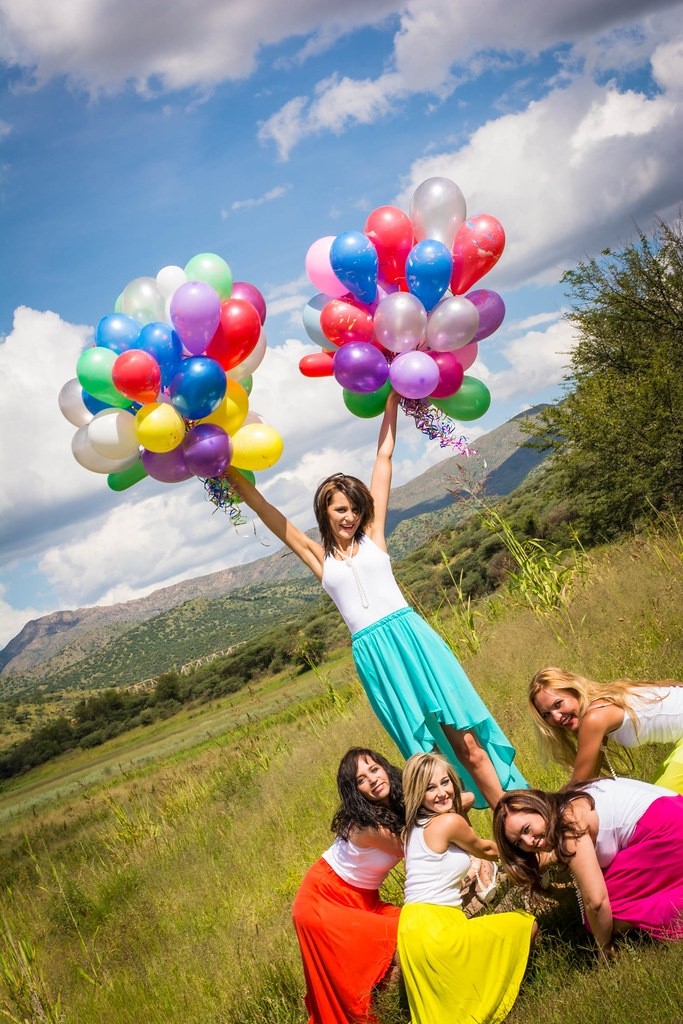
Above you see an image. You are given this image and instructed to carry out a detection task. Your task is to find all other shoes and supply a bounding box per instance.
[474,861,498,905]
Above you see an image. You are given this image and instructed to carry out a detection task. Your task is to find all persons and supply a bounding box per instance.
[397,753,538,1024]
[221,391,529,812]
[528,667,683,794]
[291,746,406,1024]
[493,778,683,947]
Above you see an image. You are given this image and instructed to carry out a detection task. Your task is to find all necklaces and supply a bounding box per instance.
[471,858,486,894]
[569,871,585,924]
[600,742,617,781]
[331,537,369,608]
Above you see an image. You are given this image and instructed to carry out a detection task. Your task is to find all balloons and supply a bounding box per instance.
[299,177,506,421]
[58,253,284,504]
